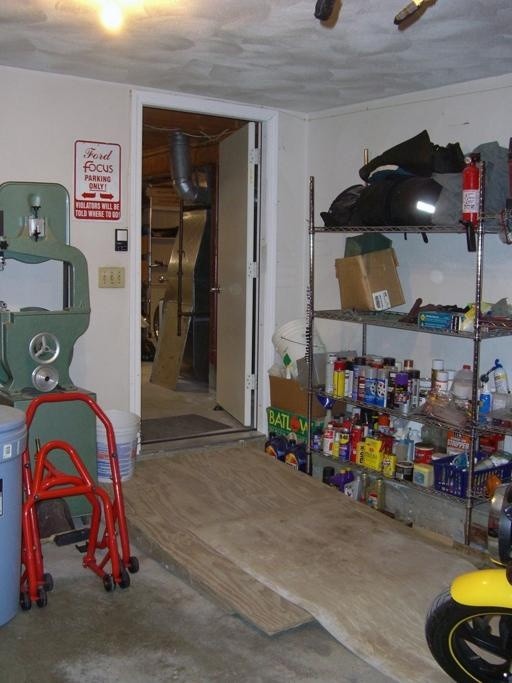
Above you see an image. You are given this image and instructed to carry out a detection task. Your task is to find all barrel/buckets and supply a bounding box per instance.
[272,318,325,379]
[94,410,142,483]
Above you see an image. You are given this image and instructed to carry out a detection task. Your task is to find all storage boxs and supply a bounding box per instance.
[265,369,319,478]
[332,248,407,318]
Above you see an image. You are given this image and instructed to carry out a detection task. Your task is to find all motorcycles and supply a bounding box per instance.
[422,476,511,683]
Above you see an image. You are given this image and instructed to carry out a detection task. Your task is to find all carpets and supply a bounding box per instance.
[143,411,232,447]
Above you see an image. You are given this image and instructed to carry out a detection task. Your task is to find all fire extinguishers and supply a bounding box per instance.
[461,152,482,228]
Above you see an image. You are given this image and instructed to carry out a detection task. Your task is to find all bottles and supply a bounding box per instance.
[312,406,365,464]
[354,473,384,511]
[325,352,344,398]
[432,358,472,408]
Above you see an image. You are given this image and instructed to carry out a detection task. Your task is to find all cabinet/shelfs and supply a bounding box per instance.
[142,185,189,357]
[300,150,511,555]
